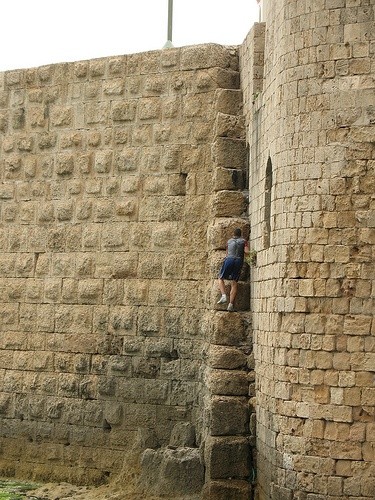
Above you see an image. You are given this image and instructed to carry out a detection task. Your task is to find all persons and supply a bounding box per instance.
[217,229,249,311]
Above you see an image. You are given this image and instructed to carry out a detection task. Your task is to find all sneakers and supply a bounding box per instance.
[217,297,234,312]
